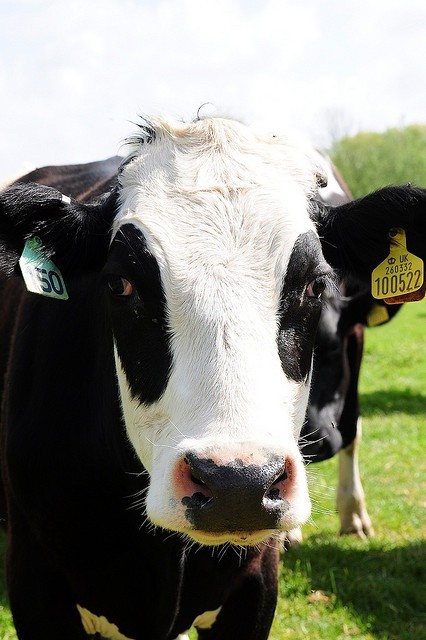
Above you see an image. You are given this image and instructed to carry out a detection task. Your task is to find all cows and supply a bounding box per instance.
[278,147,405,552]
[0,101,426,640]
